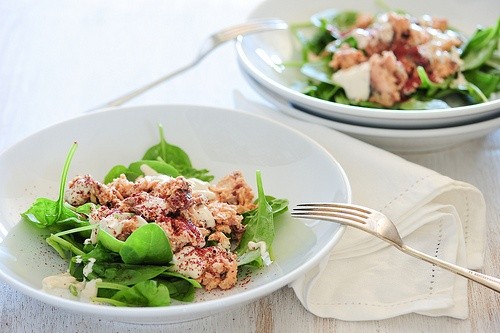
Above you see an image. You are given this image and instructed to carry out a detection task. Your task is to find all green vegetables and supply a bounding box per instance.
[276,1,500,110]
[19,124,289,307]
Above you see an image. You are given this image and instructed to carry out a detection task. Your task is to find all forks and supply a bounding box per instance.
[87,17,287,110]
[291,199,500,295]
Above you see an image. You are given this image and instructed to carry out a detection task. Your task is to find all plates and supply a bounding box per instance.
[0,102,352,324]
[232,0,499,158]
